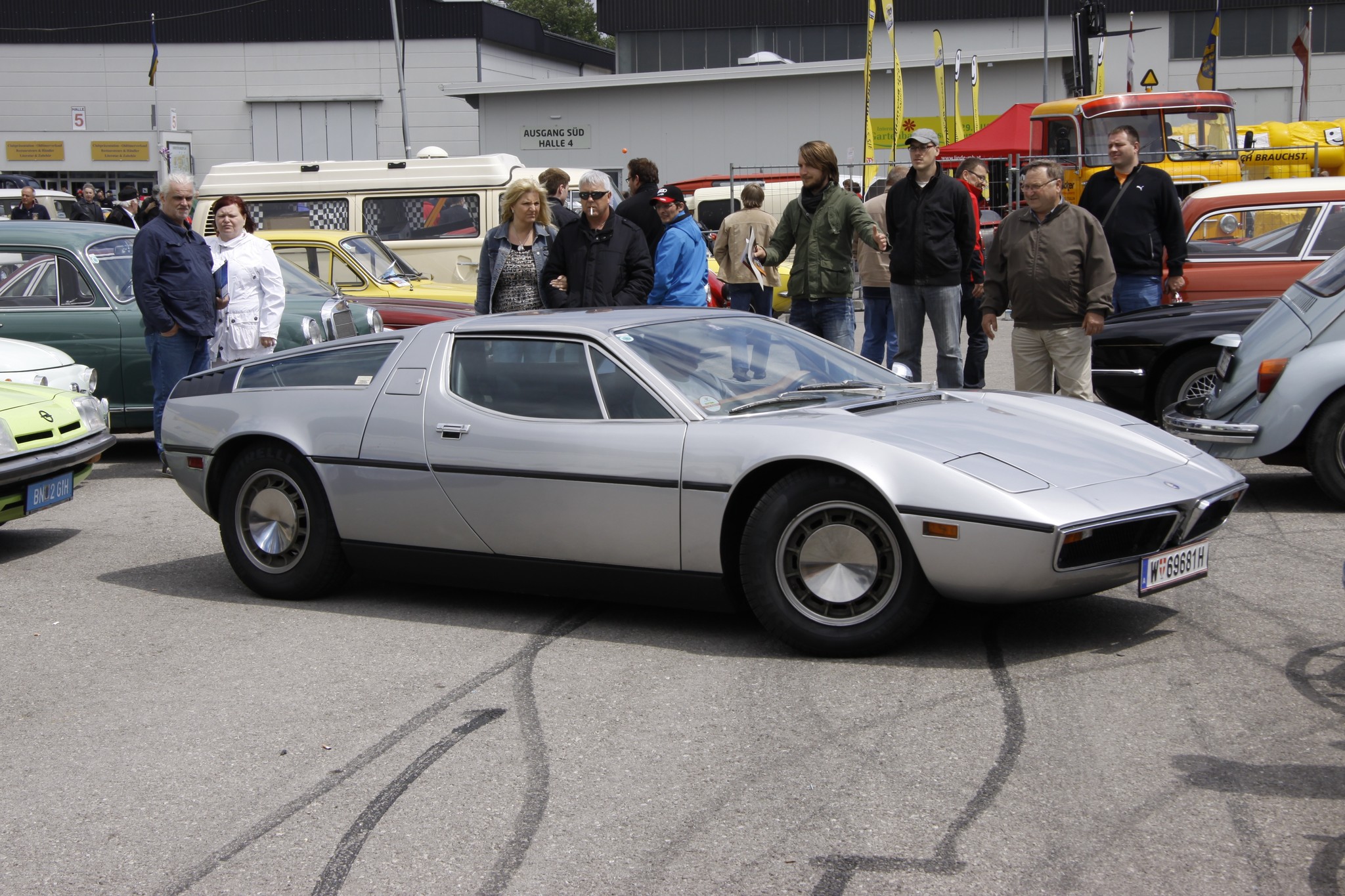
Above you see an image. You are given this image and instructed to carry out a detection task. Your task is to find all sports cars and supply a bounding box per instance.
[155,302,1255,658]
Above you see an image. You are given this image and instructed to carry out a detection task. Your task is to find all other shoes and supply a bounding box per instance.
[162,466,174,476]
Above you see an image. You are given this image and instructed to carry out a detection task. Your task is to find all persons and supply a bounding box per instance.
[982,158,1117,406]
[750,140,893,384]
[1078,125,1187,316]
[68,183,105,223]
[713,185,781,382]
[61,187,70,195]
[11,186,51,220]
[951,157,989,391]
[885,128,977,390]
[138,185,161,225]
[105,185,140,230]
[75,187,117,210]
[647,186,709,307]
[132,171,229,478]
[848,182,862,199]
[540,171,654,418]
[423,195,471,228]
[474,179,568,410]
[538,166,580,231]
[206,196,286,366]
[851,165,911,373]
[615,158,674,269]
[843,179,854,191]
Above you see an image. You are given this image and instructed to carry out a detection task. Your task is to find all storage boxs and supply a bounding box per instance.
[262,211,310,229]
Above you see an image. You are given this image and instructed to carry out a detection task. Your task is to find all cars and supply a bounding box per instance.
[0,188,479,459]
[0,333,120,533]
[703,246,796,320]
[977,80,1345,516]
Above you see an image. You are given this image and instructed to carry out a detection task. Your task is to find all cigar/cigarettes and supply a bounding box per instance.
[590,207,594,217]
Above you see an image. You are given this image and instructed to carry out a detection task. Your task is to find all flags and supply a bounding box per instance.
[1291,20,1311,122]
[1196,0,1221,91]
[1127,15,1135,91]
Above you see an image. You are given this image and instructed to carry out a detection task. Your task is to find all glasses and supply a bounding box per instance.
[908,145,935,152]
[967,170,988,183]
[1020,178,1059,193]
[626,176,635,184]
[653,202,680,210]
[579,191,609,200]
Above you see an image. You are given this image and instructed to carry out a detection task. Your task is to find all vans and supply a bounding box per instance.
[683,172,888,256]
[188,144,627,290]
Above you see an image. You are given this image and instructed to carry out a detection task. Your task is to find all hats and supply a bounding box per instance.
[152,184,160,198]
[118,186,141,201]
[904,128,939,147]
[650,185,684,204]
[106,190,113,194]
